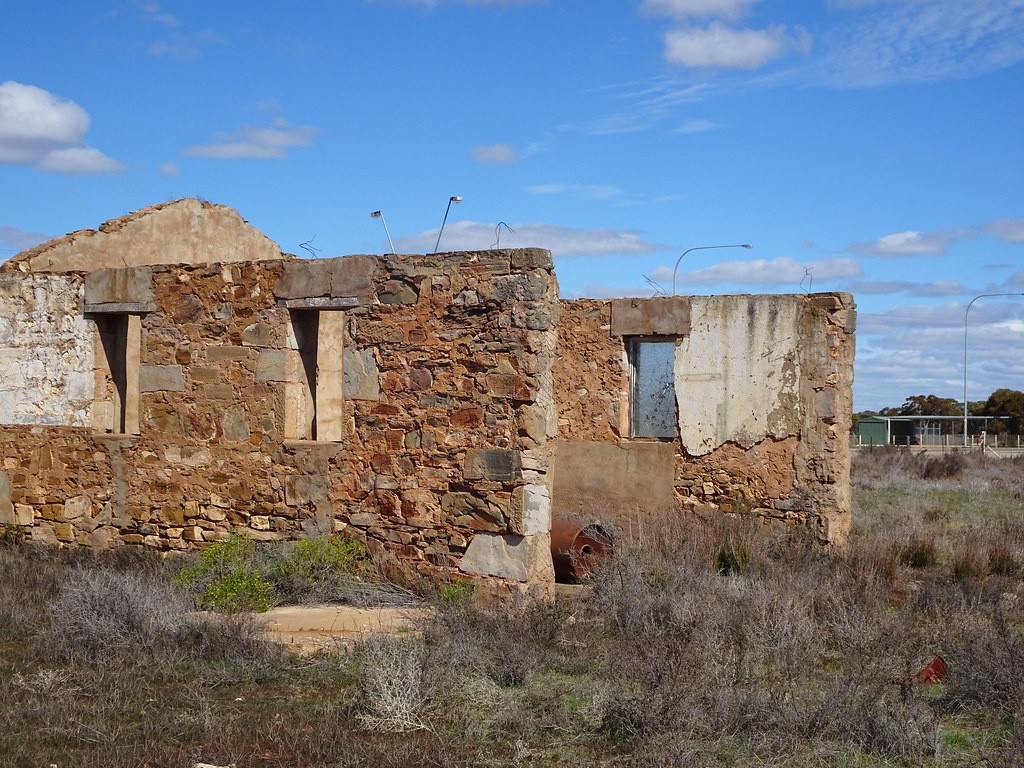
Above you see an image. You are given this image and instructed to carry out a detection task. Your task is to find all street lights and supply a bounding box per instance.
[672,246,753,295]
[370,196,463,254]
[964,293,1024,447]
